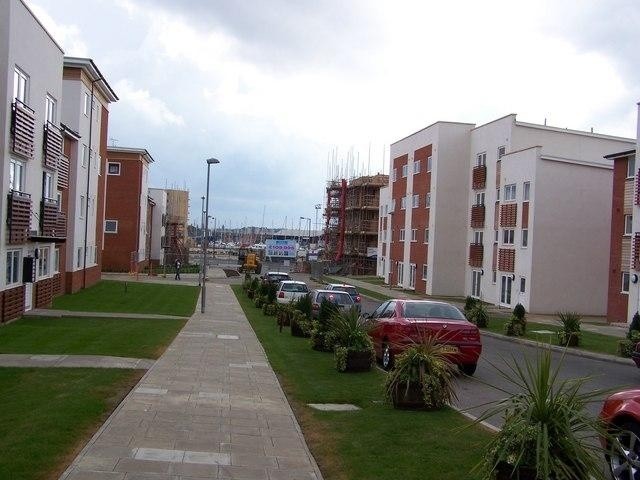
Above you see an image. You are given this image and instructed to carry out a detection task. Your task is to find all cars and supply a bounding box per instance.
[311,289,354,319]
[208,241,266,250]
[275,280,311,304]
[260,271,293,285]
[363,299,482,376]
[597,389,640,480]
[325,283,361,313]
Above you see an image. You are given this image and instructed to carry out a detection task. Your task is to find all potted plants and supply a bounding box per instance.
[553,308,585,345]
[463,295,490,329]
[323,303,383,372]
[452,331,630,480]
[244,273,339,351]
[377,313,466,411]
[503,303,527,335]
[616,311,639,357]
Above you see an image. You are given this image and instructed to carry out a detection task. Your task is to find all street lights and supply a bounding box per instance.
[300,216,311,262]
[201,158,220,313]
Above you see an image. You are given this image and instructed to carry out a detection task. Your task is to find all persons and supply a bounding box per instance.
[175,260,181,280]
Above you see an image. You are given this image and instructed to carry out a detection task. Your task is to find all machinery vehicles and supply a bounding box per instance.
[237,247,262,274]
